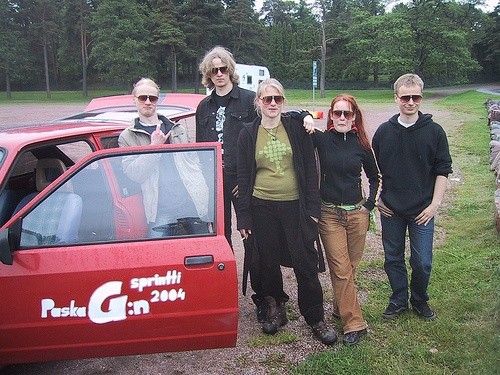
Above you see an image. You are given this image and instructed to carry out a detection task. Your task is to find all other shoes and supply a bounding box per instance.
[332,306,342,320]
[343,328,367,345]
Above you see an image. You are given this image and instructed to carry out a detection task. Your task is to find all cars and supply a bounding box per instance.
[0,93,238,365]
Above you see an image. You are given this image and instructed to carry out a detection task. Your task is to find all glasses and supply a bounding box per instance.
[331,110,354,120]
[134,95,158,104]
[258,95,284,105]
[209,67,229,75]
[396,93,423,103]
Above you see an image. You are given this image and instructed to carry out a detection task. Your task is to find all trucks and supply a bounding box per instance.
[206,63,270,96]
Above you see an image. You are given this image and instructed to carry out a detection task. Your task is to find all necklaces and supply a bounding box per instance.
[261,121,280,141]
[139,119,149,126]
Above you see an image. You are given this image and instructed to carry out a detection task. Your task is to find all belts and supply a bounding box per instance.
[321,197,367,211]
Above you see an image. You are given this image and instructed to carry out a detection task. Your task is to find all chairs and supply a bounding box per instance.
[0,189,16,228]
[9,159,83,247]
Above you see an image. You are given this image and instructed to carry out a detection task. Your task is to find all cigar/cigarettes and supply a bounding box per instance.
[240,235,248,241]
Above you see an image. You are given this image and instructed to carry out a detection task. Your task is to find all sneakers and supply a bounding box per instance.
[382,302,408,319]
[311,318,337,344]
[251,294,267,322]
[412,302,436,320]
[262,296,288,334]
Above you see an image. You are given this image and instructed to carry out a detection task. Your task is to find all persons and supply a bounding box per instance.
[237,76,337,344]
[295,93,383,348]
[119,78,214,236]
[196,47,266,324]
[371,74,453,321]
